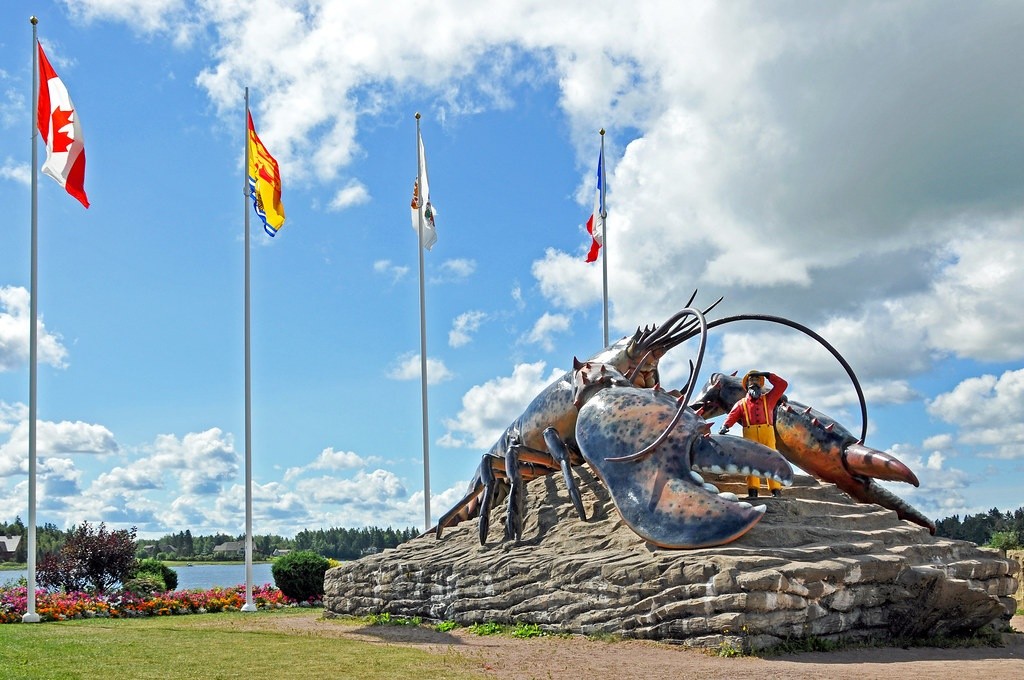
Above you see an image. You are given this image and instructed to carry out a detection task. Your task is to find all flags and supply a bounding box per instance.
[248,107,285,237]
[34,36,92,210]
[408,128,439,253]
[585,144,608,263]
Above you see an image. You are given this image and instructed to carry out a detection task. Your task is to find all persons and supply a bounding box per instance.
[718,370,789,499]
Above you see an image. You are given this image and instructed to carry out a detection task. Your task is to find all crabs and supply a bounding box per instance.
[689,370,940,538]
[417,287,869,551]
